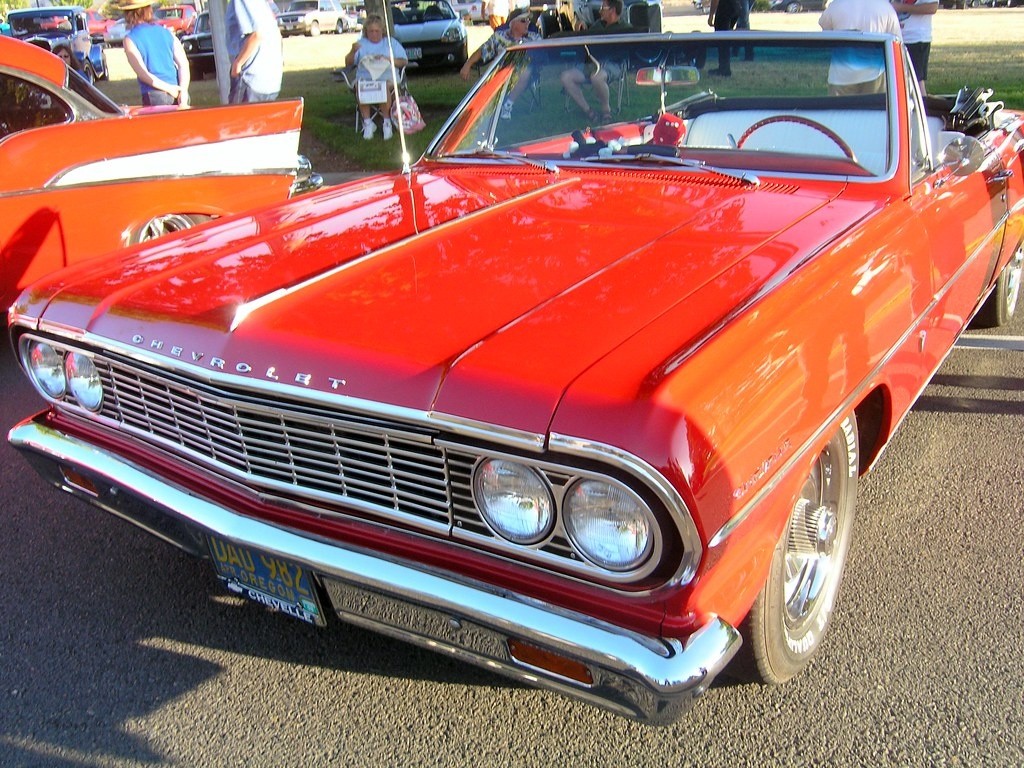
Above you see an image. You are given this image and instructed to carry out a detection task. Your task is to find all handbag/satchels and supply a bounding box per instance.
[390,96,426,136]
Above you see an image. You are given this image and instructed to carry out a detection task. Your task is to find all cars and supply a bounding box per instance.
[7,31,1024,726]
[769,0,830,13]
[0,37,305,309]
[153,5,200,39]
[0,22,12,37]
[41,10,115,42]
[181,10,217,80]
[8,5,106,86]
[103,18,131,43]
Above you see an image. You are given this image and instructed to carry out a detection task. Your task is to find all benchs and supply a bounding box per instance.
[645,109,943,178]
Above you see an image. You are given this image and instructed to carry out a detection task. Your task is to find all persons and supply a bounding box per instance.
[225,0,283,104]
[818,0,903,97]
[707,0,743,76]
[51,43,89,108]
[561,0,633,124]
[115,0,191,107]
[888,0,939,96]
[459,6,546,120]
[345,12,408,141]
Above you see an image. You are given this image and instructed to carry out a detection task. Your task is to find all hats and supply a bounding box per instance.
[111,0,158,11]
[505,8,528,25]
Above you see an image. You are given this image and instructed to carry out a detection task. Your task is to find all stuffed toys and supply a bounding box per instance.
[563,130,622,159]
[644,113,690,146]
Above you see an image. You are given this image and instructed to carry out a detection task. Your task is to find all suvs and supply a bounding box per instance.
[276,0,348,38]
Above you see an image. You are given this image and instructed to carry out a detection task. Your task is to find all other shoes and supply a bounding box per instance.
[600,110,614,125]
[707,68,732,76]
[585,106,598,124]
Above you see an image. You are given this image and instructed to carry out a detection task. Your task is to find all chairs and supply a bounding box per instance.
[329,59,418,133]
[562,59,631,117]
[476,56,542,111]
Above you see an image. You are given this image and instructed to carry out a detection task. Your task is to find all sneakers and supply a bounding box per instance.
[500,104,513,121]
[383,123,393,141]
[360,121,377,141]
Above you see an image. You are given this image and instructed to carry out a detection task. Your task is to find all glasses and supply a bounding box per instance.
[600,5,610,11]
[520,17,531,23]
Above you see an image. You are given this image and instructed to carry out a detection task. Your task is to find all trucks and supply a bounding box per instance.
[364,0,469,74]
